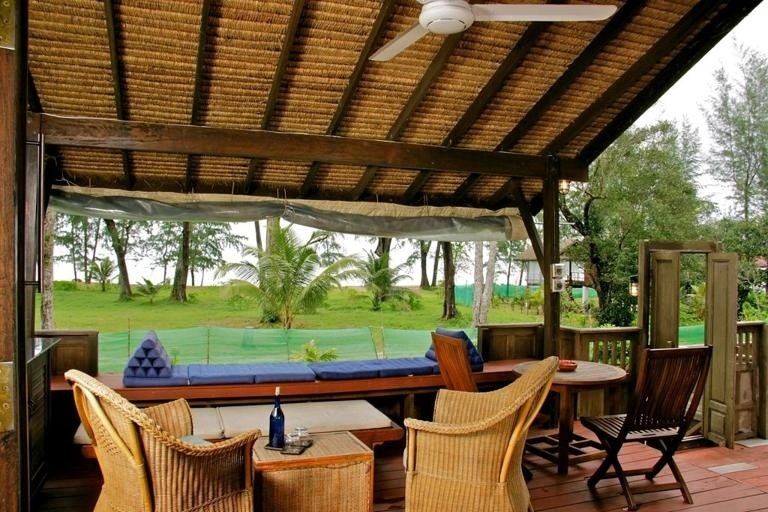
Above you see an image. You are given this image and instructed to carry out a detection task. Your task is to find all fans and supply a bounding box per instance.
[366,0,619,64]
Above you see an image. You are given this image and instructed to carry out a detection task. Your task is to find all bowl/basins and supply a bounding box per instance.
[559,361,577,371]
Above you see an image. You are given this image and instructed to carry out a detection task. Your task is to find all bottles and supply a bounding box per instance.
[269,386,285,448]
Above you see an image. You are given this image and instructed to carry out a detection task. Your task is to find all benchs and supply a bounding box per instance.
[49,356,542,433]
[74,400,406,461]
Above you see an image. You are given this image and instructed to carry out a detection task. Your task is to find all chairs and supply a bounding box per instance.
[578,345,713,510]
[431,332,538,482]
[401,356,559,512]
[62,368,262,511]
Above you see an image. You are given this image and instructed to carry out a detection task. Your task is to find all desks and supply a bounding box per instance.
[511,359,627,473]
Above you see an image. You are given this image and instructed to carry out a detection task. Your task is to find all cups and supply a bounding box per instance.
[294,426,310,445]
[284,432,301,452]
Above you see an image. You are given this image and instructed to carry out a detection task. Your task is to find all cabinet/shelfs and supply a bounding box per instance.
[24,335,63,498]
